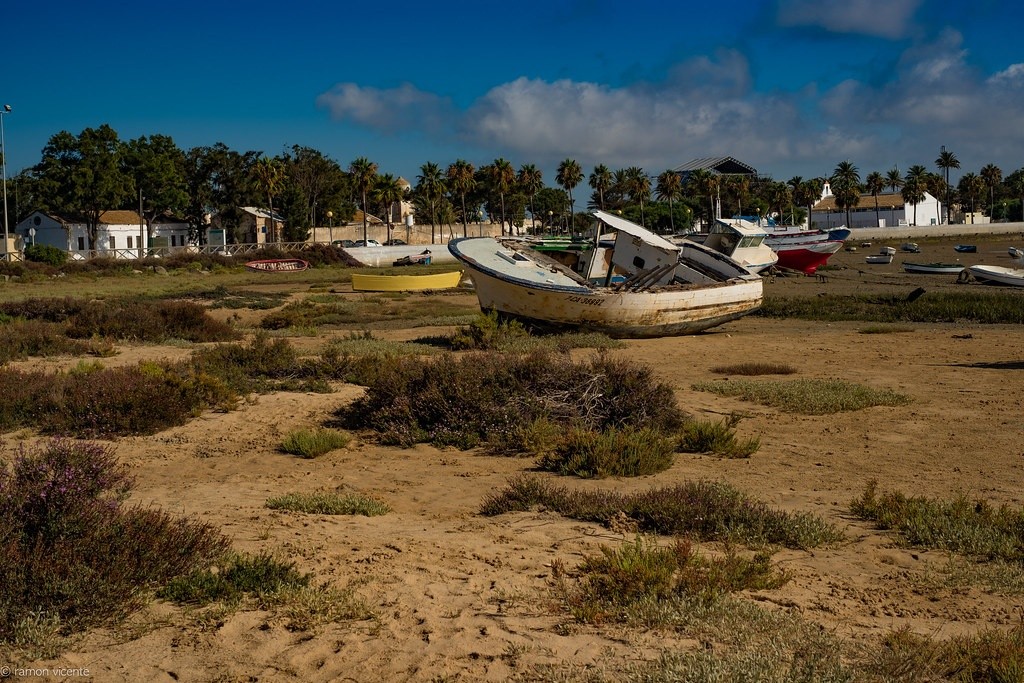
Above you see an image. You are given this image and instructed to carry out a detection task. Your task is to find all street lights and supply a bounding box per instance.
[478,210,483,237]
[327,211,334,245]
[756,208,761,227]
[686,208,691,231]
[548,211,553,236]
[404,211,410,245]
[0,104,12,266]
[826,206,830,229]
[1003,202,1007,222]
[891,205,896,227]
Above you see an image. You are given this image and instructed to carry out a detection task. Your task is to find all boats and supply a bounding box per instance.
[243,259,310,273]
[902,261,967,273]
[901,242,919,252]
[954,244,977,253]
[392,248,434,268]
[1008,246,1024,259]
[969,265,1024,286]
[350,269,465,292]
[447,208,897,340]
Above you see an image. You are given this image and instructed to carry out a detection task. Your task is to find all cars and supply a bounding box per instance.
[356,239,383,247]
[332,239,359,248]
[381,239,408,245]
